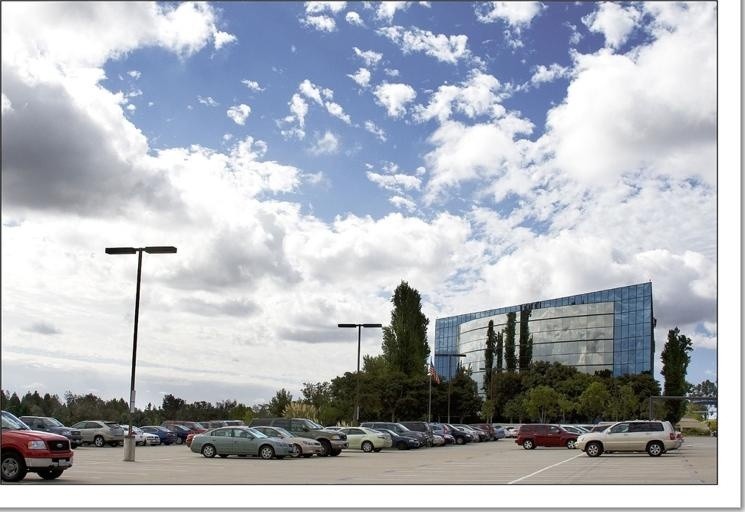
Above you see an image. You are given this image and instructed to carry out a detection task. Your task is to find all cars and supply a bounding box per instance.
[123,425,161,446]
[561,421,629,434]
[69,419,124,447]
[190,425,291,460]
[335,426,393,453]
[162,419,245,445]
[360,421,520,450]
[244,426,324,459]
[140,425,177,446]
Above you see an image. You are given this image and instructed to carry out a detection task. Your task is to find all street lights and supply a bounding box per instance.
[338,324,385,424]
[434,354,465,422]
[104,245,177,463]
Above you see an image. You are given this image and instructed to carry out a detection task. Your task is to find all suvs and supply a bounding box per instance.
[1,411,74,481]
[515,424,580,450]
[247,417,348,458]
[18,415,84,449]
[577,419,682,457]
